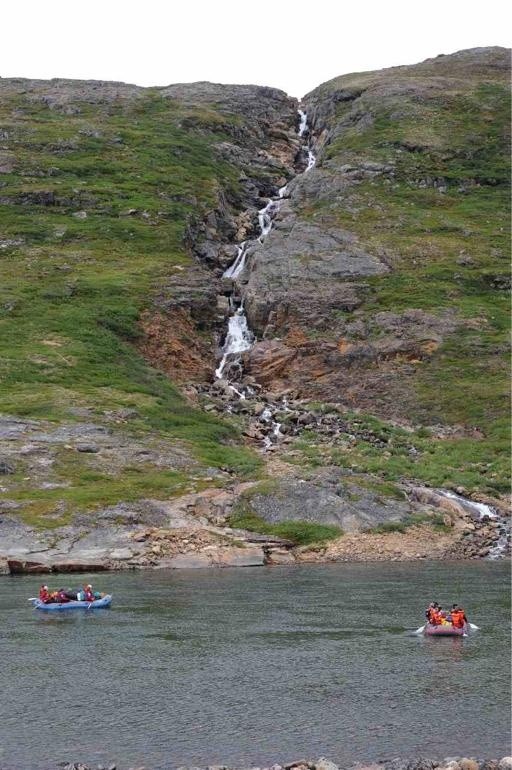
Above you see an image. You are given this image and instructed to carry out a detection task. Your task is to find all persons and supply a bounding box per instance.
[425,601,468,629]
[39,584,96,603]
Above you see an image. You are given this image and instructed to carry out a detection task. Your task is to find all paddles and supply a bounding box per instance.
[468,623,479,629]
[415,619,432,634]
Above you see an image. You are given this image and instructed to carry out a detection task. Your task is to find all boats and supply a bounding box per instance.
[32,595,112,610]
[424,625,464,636]
